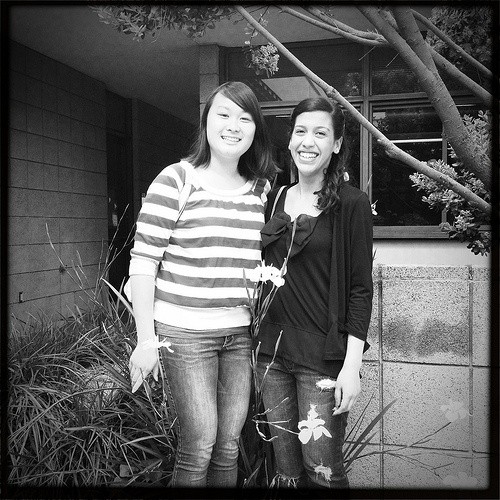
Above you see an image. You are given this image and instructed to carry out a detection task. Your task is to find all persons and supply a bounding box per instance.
[250,96,377,487]
[125,80,282,486]
